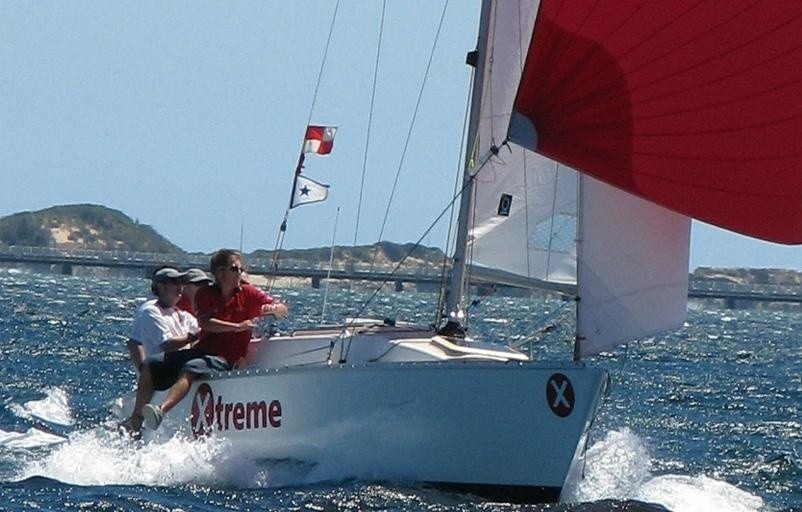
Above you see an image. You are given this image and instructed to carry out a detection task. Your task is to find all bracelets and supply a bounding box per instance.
[187,331,196,341]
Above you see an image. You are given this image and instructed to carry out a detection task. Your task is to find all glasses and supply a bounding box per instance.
[226,265,244,272]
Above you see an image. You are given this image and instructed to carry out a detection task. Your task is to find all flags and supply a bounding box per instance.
[302,125,338,162]
[291,175,330,210]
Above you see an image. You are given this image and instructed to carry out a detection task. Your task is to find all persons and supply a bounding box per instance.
[138,269,202,361]
[176,269,213,314]
[128,264,176,368]
[241,271,251,284]
[120,248,287,439]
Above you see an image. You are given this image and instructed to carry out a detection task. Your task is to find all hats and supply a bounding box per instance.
[183,268,214,283]
[151,268,190,284]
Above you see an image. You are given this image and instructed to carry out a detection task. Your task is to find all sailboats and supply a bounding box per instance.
[109,1,609,504]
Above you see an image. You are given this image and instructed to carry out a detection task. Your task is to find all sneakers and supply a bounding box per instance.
[104,420,141,440]
[143,404,163,430]
